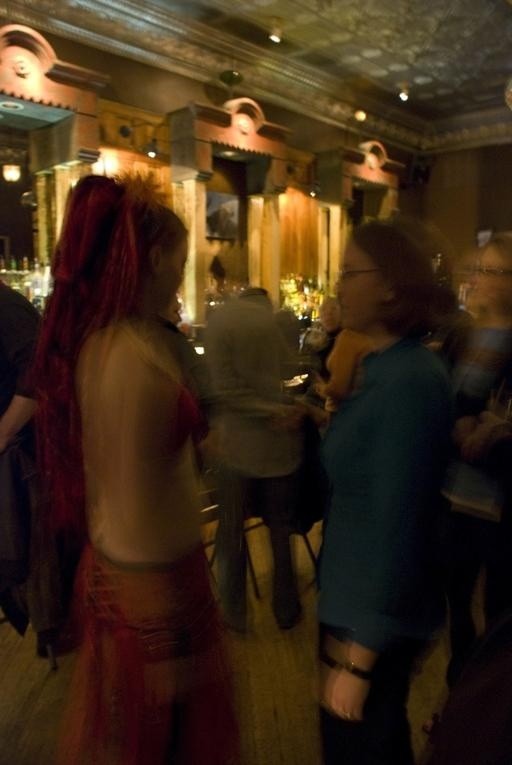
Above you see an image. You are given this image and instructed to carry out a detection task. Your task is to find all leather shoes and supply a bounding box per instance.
[271,565,303,630]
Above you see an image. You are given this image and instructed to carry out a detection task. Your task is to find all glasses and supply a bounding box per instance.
[341,267,382,281]
[479,266,512,276]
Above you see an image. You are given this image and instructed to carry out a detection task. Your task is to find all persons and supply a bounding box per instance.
[203,282,313,628]
[0,283,43,642]
[32,174,243,765]
[310,215,466,765]
[422,234,512,764]
[313,295,382,408]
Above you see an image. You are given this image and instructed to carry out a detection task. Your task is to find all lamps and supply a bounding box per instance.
[119,116,166,159]
[344,109,367,146]
[214,70,241,106]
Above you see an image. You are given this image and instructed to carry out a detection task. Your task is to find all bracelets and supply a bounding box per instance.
[344,660,372,680]
